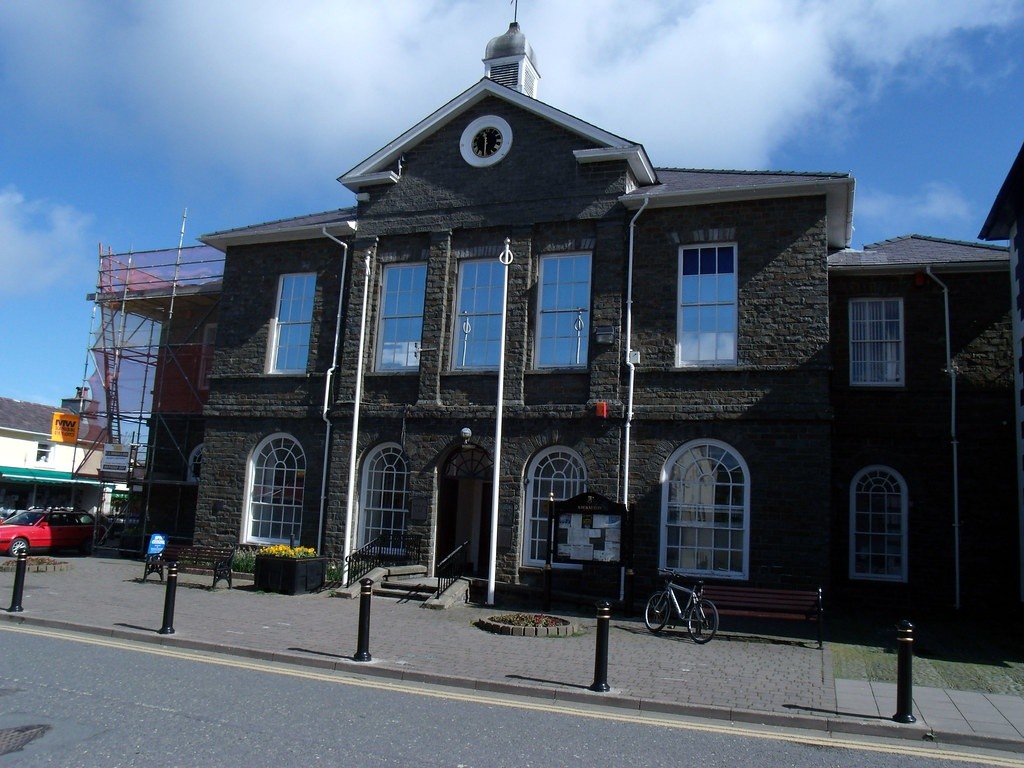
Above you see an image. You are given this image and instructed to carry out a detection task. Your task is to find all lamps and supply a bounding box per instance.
[461,428,474,449]
[595,326,615,345]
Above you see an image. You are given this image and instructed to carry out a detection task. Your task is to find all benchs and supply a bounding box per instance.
[142,543,236,590]
[694,581,825,649]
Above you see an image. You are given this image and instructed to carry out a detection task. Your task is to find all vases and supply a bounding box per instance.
[253,554,327,595]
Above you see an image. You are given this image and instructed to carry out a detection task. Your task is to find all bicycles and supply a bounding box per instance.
[646,568,718,645]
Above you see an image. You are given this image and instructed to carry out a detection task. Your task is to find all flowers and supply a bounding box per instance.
[259,544,317,558]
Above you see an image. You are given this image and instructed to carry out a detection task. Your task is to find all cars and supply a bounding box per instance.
[0,511,108,557]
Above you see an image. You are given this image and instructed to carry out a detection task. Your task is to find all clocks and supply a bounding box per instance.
[471,126,504,158]
